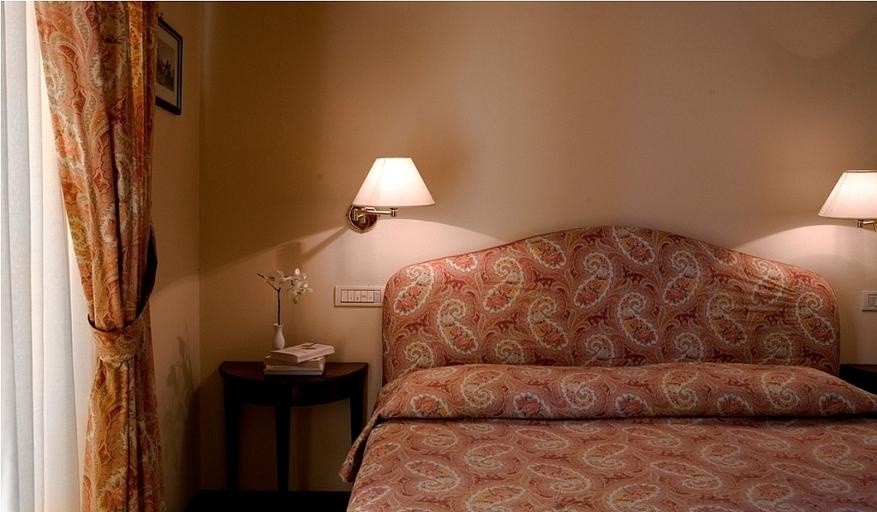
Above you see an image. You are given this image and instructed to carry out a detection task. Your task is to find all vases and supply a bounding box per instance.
[272,323,285,350]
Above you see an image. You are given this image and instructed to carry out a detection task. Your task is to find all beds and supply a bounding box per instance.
[346,223,877,511]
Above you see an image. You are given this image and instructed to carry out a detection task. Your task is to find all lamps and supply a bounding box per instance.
[346,156,436,234]
[819,168,876,228]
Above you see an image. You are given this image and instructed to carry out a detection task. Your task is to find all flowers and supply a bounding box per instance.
[256,268,313,325]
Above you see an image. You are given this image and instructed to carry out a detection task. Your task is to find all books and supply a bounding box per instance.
[263,342,335,376]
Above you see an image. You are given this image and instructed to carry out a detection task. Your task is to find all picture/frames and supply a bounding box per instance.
[154,14,185,116]
